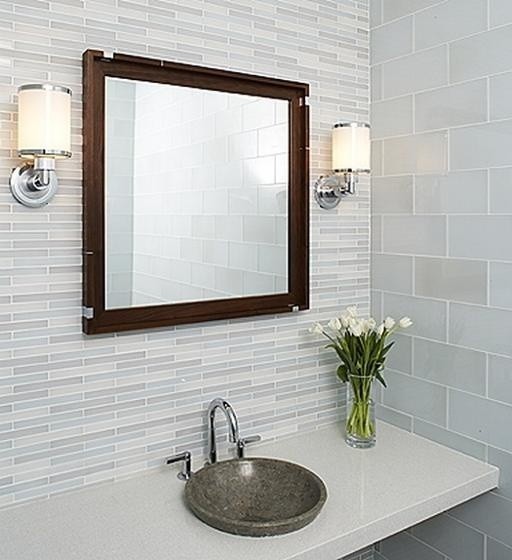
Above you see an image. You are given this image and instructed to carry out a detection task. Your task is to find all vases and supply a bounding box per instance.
[345,372,377,450]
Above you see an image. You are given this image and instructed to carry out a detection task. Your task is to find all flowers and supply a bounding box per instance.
[310,306,413,440]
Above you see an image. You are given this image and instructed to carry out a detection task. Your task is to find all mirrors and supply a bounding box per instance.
[80,48,310,333]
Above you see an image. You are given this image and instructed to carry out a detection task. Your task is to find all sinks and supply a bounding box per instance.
[185,457,328,537]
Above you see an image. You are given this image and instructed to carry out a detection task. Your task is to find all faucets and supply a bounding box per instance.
[207,398,239,465]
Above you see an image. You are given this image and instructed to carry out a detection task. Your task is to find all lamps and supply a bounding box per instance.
[314,121,372,210]
[10,83,73,209]
